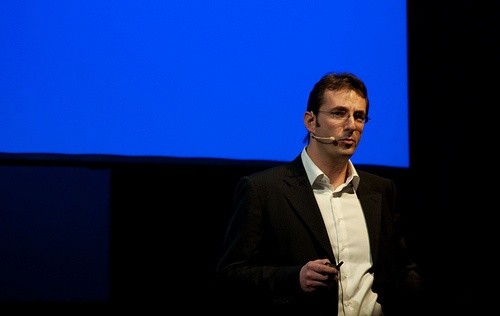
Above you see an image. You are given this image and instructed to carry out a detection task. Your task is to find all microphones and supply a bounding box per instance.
[310,132,335,141]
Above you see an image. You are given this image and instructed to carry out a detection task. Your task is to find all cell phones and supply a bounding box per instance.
[320,261,343,281]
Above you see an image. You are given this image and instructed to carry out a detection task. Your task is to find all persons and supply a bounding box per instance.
[216,72,421,316]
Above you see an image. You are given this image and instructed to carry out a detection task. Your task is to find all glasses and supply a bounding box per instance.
[309,109,369,124]
